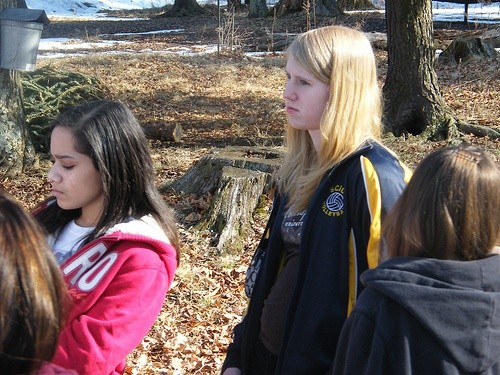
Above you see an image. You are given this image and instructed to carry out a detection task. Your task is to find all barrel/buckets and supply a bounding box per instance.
[0,20,43,72]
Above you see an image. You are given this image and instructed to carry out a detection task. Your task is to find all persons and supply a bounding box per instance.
[221,25,413,375]
[332,144,500,375]
[27,100,179,375]
[0,194,71,375]
